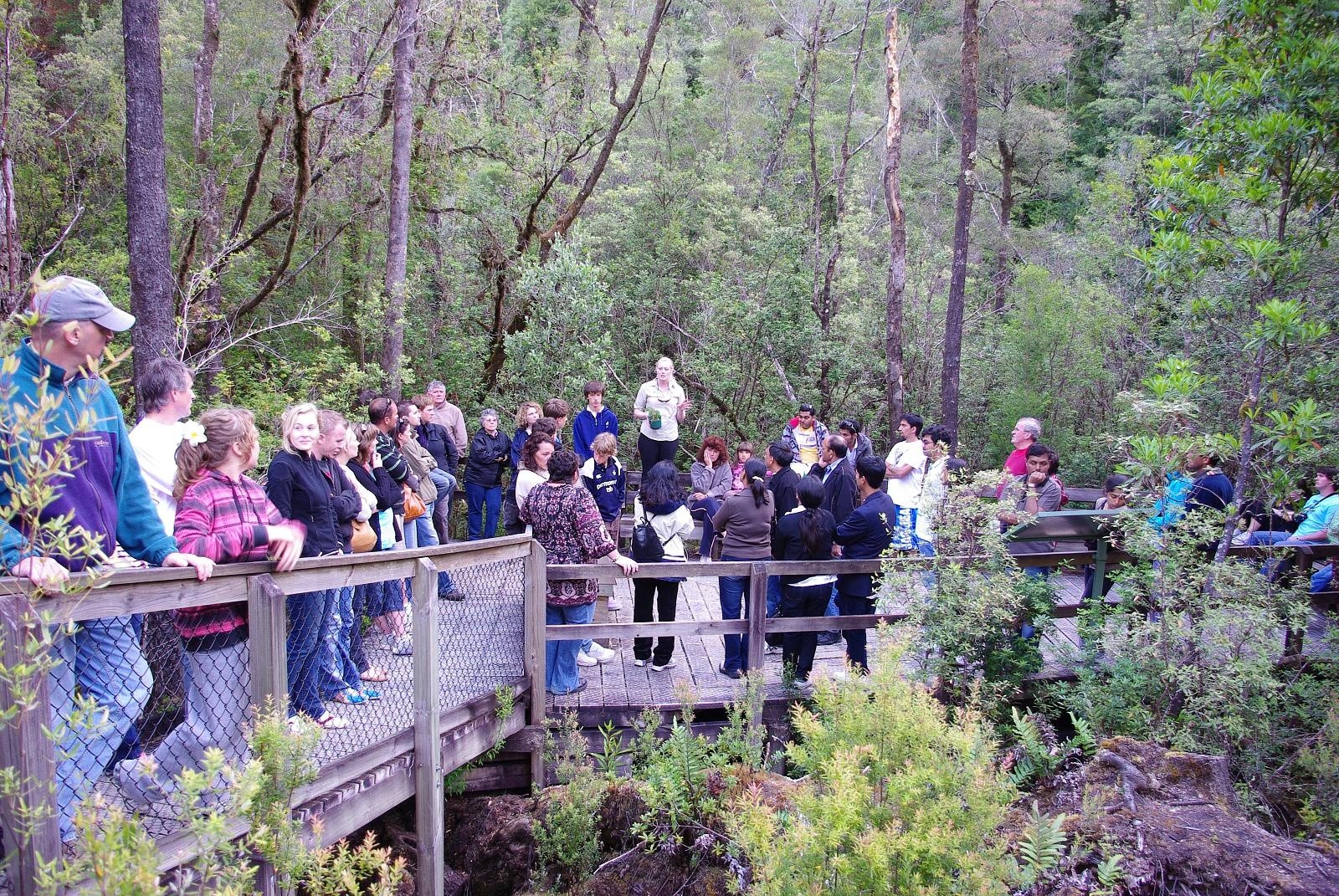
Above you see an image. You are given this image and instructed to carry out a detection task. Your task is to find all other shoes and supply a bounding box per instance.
[609,599,622,610]
[113,760,122,786]
[361,668,385,681]
[700,556,711,562]
[381,630,396,645]
[317,716,345,729]
[1231,532,1250,545]
[1070,650,1104,663]
[719,661,741,678]
[393,635,414,655]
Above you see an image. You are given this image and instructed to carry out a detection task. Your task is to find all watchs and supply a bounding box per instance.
[1296,536,1299,541]
[1028,483,1037,488]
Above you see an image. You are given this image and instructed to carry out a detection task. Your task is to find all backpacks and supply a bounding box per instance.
[632,510,676,561]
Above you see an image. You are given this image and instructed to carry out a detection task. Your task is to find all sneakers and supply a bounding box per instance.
[548,642,676,694]
[359,687,381,699]
[443,588,465,600]
[817,630,842,645]
[794,678,813,690]
[764,643,783,654]
[331,688,364,704]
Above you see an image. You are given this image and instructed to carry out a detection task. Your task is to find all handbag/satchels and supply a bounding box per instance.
[351,519,377,552]
[400,482,426,523]
[765,607,784,646]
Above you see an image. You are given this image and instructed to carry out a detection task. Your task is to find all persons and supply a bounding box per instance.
[0,276,217,869]
[99,356,1339,814]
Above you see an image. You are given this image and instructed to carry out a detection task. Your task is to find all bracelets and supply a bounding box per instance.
[705,494,709,498]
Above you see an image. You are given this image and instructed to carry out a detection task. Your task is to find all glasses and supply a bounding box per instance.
[842,421,856,432]
[382,396,391,419]
[657,389,671,402]
[801,404,812,409]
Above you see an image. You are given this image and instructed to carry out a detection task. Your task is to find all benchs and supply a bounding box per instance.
[622,472,713,539]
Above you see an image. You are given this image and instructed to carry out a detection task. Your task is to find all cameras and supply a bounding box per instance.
[1294,514,1307,522]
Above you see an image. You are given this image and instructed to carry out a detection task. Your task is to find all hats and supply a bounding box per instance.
[25,275,135,332]
